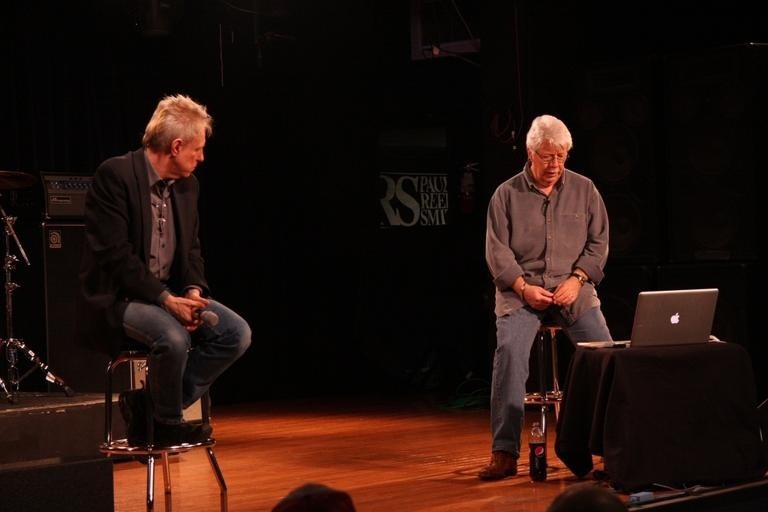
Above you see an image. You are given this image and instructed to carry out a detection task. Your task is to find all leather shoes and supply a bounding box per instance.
[478,453,517,479]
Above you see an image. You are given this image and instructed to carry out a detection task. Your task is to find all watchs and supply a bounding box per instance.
[568,272,588,287]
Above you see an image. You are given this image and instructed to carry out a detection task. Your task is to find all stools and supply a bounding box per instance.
[522,307,566,465]
[91,346,228,512]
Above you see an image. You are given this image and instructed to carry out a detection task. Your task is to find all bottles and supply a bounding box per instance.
[528,421,548,480]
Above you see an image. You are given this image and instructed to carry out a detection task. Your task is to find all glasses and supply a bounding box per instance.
[532,148,570,162]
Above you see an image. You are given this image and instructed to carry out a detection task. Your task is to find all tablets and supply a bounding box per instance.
[630,288,718,347]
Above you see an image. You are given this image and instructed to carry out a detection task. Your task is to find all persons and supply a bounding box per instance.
[477,111,615,480]
[81,93,254,464]
[546,482,625,510]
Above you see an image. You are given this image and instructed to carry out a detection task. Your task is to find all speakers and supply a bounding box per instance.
[40,222,128,393]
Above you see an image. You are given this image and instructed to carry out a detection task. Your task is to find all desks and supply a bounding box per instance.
[554,335,767,490]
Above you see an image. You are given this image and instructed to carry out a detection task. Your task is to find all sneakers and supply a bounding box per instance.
[154,421,210,444]
[118,379,155,465]
[494,474,498,476]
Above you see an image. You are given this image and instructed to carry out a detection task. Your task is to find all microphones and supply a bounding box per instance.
[193,307,219,327]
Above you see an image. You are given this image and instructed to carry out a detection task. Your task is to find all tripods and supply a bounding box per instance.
[0,215,76,405]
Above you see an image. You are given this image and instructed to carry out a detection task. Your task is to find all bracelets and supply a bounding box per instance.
[520,280,526,305]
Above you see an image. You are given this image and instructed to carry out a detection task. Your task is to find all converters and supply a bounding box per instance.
[631,491,654,502]
